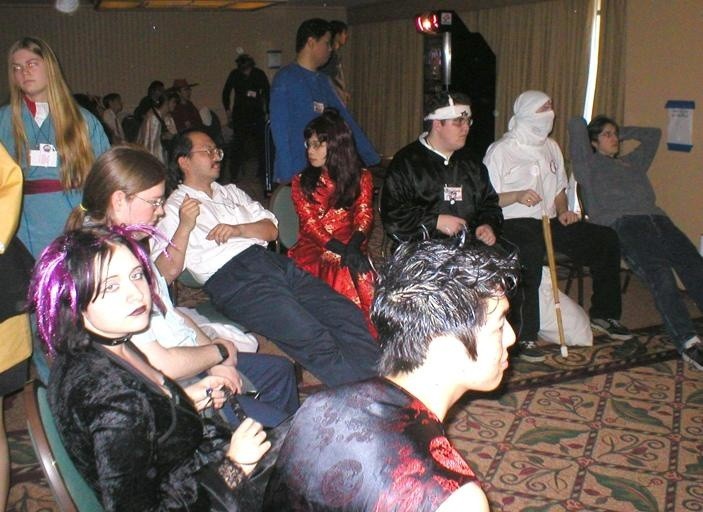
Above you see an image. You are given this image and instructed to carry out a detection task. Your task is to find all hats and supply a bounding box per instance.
[168,78,199,91]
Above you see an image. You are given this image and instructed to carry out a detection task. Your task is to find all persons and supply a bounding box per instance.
[381,92,530,359]
[481,91,634,363]
[26,225,283,512]
[0,36,111,259]
[261,236,525,512]
[0,144,36,512]
[567,114,703,369]
[72,18,387,433]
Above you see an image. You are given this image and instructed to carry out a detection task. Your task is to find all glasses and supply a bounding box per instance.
[302,138,326,151]
[133,194,167,210]
[441,116,474,128]
[188,146,224,162]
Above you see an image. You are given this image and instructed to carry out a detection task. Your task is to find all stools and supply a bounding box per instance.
[543,251,583,305]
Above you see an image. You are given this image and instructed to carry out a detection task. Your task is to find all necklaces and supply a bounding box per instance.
[426,137,436,151]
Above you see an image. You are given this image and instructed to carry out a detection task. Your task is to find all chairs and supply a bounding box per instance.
[24,379,102,511]
[171,268,305,385]
[378,183,466,260]
[565,182,633,296]
[269,184,300,256]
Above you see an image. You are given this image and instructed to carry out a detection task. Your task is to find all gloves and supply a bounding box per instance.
[325,230,374,274]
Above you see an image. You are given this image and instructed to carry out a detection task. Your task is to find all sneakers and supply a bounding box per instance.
[510,340,546,362]
[590,316,634,341]
[681,342,703,371]
